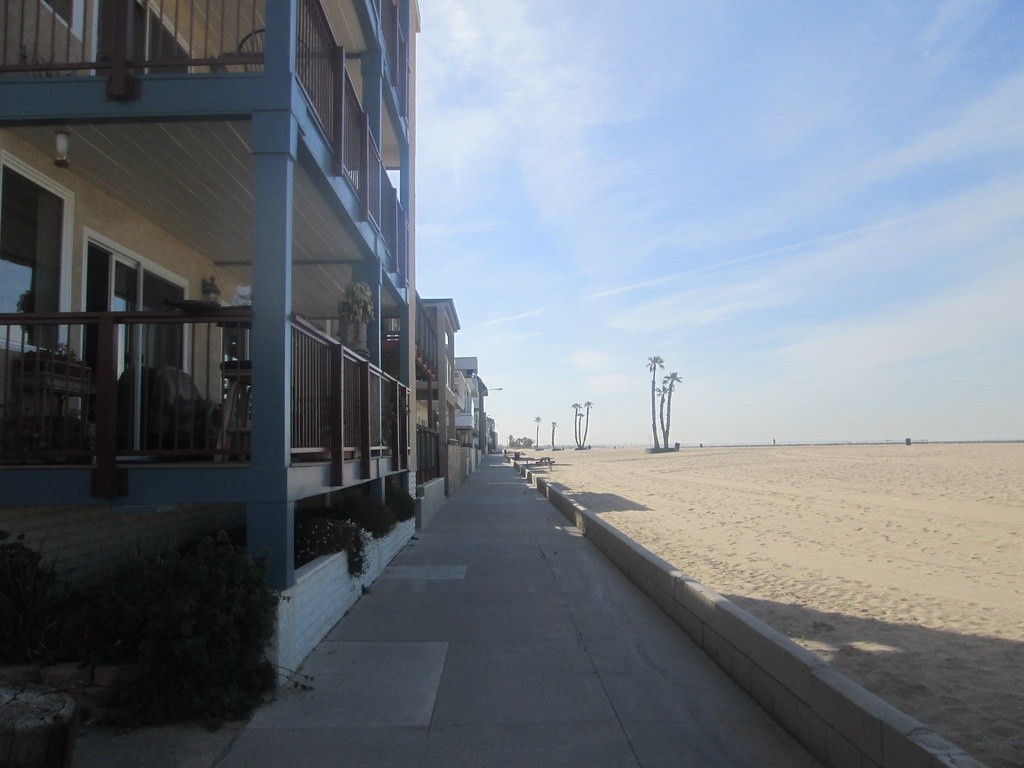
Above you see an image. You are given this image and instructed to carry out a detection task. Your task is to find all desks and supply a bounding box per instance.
[540,457,550,466]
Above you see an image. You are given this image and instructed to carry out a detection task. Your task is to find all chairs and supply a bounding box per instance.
[158,366,221,462]
[23,44,73,78]
[239,28,311,80]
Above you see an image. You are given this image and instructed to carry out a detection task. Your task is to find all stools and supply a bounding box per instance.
[213,360,251,464]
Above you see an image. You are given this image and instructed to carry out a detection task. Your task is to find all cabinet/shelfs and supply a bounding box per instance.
[12,357,91,465]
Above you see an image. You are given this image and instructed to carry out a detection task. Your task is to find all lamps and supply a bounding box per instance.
[202,273,221,303]
[54,125,72,168]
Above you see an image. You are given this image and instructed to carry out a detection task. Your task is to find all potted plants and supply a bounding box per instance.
[338,281,375,359]
